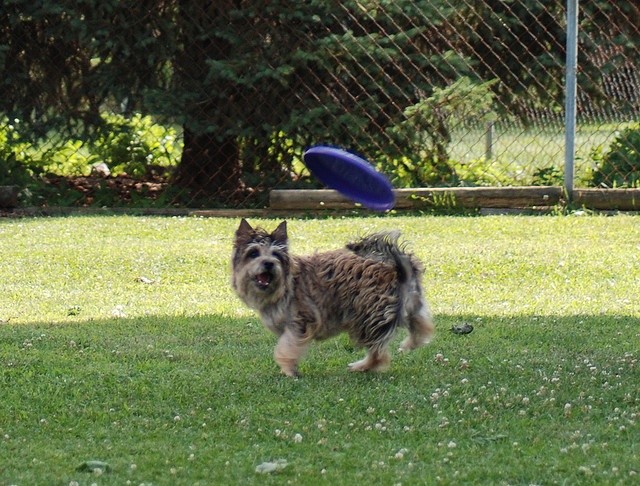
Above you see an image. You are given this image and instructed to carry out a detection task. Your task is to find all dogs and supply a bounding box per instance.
[231,217,435,378]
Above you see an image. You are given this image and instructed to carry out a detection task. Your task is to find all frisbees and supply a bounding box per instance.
[304,146,396,211]
